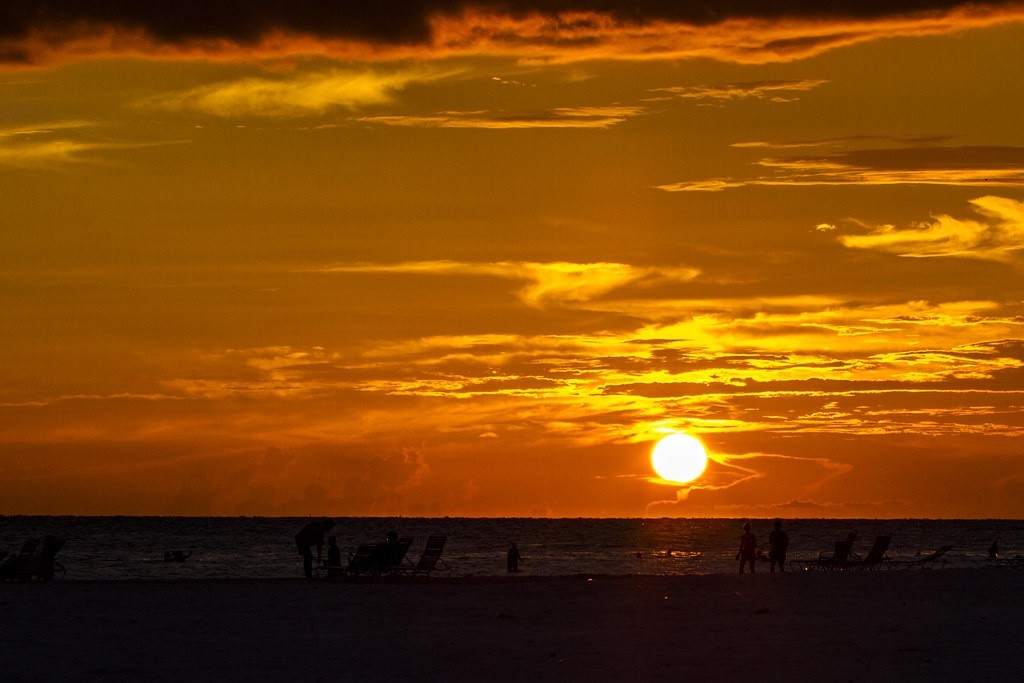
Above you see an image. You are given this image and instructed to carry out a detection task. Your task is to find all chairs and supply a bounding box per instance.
[981,555,1024,566]
[889,543,956,571]
[343,541,384,579]
[817,535,891,573]
[30,535,72,581]
[0,538,40,584]
[399,534,452,578]
[789,530,860,573]
[314,565,347,578]
[367,536,415,577]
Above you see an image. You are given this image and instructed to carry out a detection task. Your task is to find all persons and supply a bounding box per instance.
[386,531,398,543]
[767,520,790,573]
[295,517,337,580]
[324,536,343,579]
[505,541,524,573]
[735,522,759,576]
